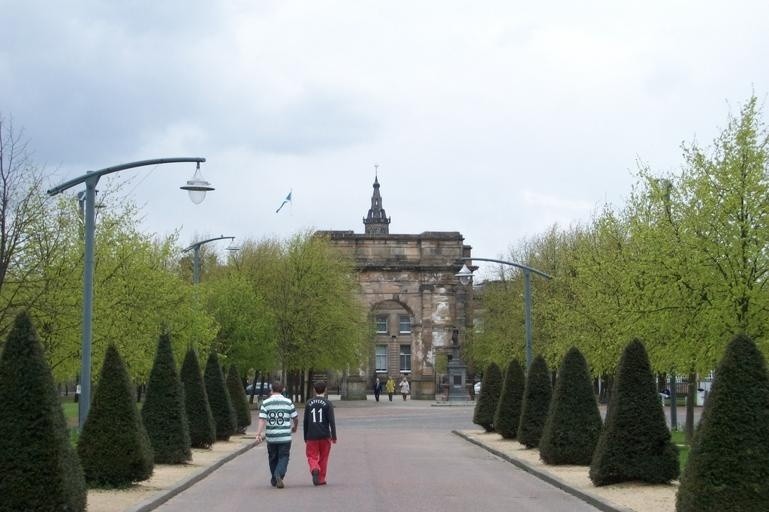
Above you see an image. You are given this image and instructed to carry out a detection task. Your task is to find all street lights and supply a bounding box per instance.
[45,154,213,428]
[451,256,554,365]
[180,235,239,356]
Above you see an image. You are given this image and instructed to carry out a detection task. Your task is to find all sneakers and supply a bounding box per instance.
[313,468,320,486]
[271,472,284,488]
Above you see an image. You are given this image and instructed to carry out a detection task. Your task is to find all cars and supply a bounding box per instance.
[473,381,481,395]
[246,382,273,396]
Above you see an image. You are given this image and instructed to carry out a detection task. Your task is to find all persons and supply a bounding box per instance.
[398,376,410,402]
[253,379,300,489]
[373,377,383,402]
[385,375,396,402]
[302,379,338,488]
